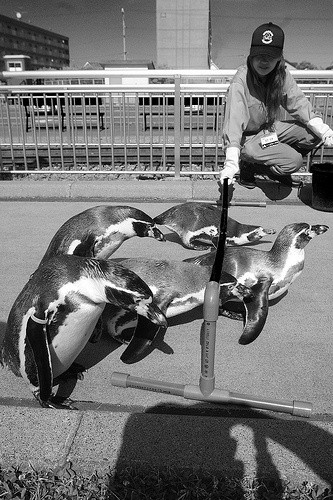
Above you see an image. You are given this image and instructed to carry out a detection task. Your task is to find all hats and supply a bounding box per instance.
[250,22,284,58]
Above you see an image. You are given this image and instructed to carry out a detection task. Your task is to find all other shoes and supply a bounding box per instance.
[239,161,256,189]
[268,172,296,186]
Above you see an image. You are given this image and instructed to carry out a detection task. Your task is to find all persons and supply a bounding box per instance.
[220,21,333,189]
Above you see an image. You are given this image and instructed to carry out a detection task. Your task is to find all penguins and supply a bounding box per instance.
[5,254,169,411]
[151,201,277,250]
[102,258,256,343]
[45,205,165,259]
[182,221,330,345]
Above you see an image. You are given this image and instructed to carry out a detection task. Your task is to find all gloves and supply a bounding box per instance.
[219,147,241,185]
[307,117,333,146]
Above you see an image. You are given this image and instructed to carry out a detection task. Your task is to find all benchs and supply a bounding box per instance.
[139,97,222,131]
[23,98,105,132]
